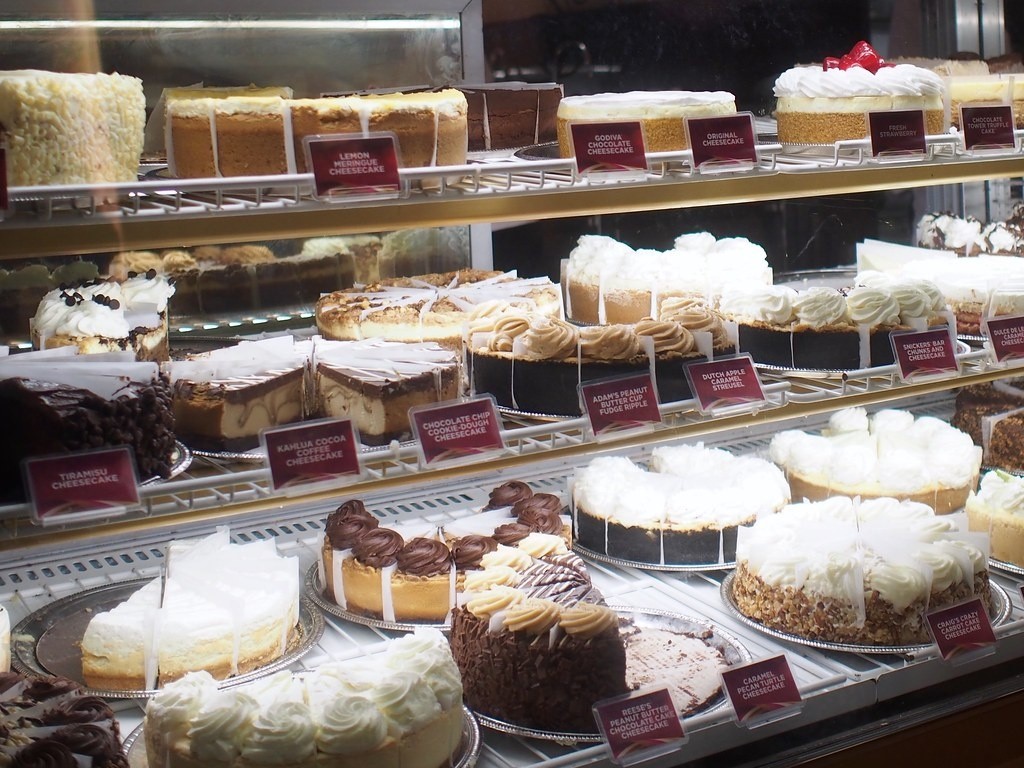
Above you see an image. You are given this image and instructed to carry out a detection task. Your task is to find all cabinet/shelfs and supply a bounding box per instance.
[0,128,1024,768]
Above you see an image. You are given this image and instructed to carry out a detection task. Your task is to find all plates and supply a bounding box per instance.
[3,139,1023,766]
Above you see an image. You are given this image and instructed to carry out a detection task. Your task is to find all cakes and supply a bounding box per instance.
[0,38,1024,196]
[0,374,1024,768]
[0,208,1024,507]
